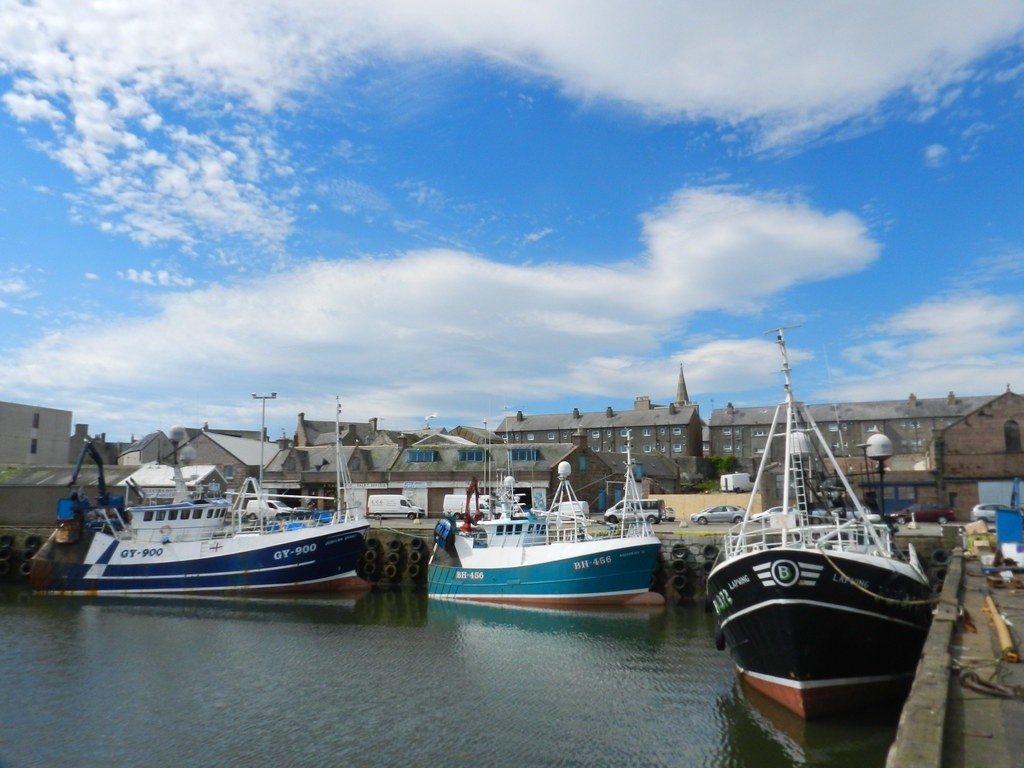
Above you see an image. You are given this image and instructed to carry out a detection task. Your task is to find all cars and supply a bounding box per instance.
[829,506,870,522]
[883,501,955,526]
[970,504,1023,522]
[750,506,802,524]
[798,507,828,524]
[690,505,747,525]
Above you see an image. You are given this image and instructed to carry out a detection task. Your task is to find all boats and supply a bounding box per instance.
[428,407,657,602]
[28,437,373,594]
[702,322,931,721]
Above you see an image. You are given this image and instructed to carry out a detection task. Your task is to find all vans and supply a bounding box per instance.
[547,500,589,521]
[720,473,755,493]
[246,500,293,520]
[603,499,666,525]
[442,495,497,520]
[368,494,425,520]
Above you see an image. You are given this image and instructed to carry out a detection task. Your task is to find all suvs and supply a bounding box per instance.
[664,507,675,522]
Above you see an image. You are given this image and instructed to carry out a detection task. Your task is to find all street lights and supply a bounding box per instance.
[251,391,277,501]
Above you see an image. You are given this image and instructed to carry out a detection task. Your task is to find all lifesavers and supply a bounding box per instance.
[160,524,172,536]
[930,547,951,595]
[671,542,688,591]
[363,537,380,575]
[898,548,922,562]
[384,539,403,579]
[19,533,41,577]
[0,534,14,577]
[407,538,424,578]
[701,544,719,585]
